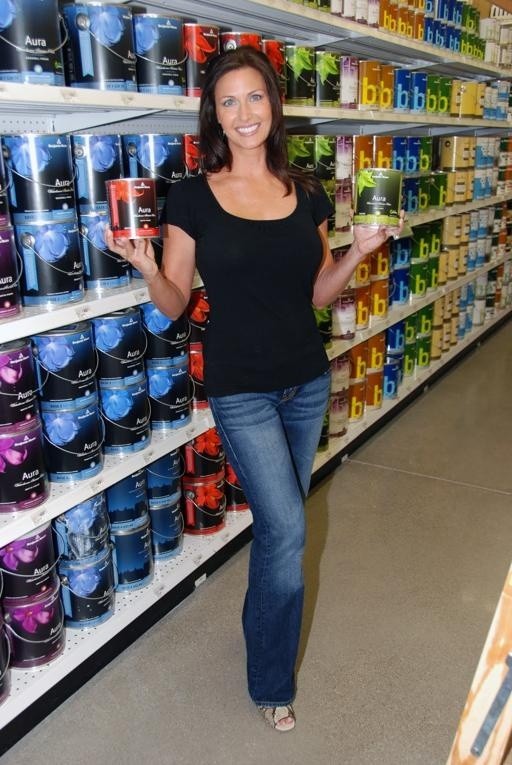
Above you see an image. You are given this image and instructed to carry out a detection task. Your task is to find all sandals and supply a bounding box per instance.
[258,704,296,732]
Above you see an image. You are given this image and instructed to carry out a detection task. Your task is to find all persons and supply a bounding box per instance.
[104,43,406,733]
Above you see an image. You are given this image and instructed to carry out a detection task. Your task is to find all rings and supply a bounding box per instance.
[391,228,400,240]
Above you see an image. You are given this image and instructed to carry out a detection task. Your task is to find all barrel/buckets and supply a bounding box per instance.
[286,134,352,237]
[79,213,130,291]
[105,178,161,239]
[147,358,195,430]
[54,490,110,568]
[316,259,512,453]
[0,615,11,709]
[261,39,287,104]
[286,45,512,121]
[105,467,149,534]
[29,320,99,412]
[353,133,434,174]
[100,378,153,456]
[133,13,183,96]
[438,135,512,207]
[62,1,137,92]
[403,173,447,218]
[147,447,186,509]
[91,307,148,389]
[180,426,226,485]
[70,133,123,215]
[312,220,442,350]
[0,424,51,514]
[15,218,85,307]
[188,351,210,409]
[293,0,512,72]
[184,133,205,177]
[121,133,187,209]
[2,575,65,668]
[187,288,209,353]
[182,478,227,535]
[0,339,40,435]
[2,133,77,223]
[42,400,104,484]
[0,520,65,605]
[139,301,191,368]
[57,549,119,628]
[131,211,163,279]
[149,503,185,562]
[0,0,68,86]
[225,463,250,512]
[220,32,262,52]
[110,517,153,593]
[0,226,24,320]
[353,168,402,227]
[438,199,512,285]
[0,139,11,229]
[183,23,220,96]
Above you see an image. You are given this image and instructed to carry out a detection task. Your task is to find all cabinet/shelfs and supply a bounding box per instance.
[0,1,512,764]
